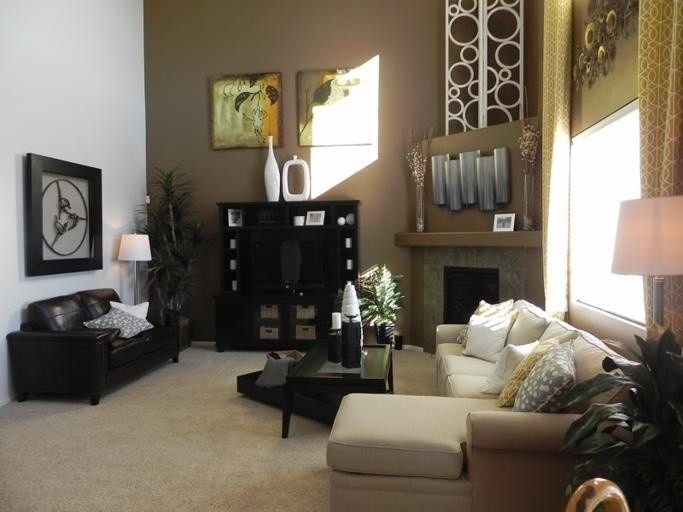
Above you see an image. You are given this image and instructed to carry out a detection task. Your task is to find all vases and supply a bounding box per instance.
[415,186,424,233]
[522,174,533,232]
[263,135,282,202]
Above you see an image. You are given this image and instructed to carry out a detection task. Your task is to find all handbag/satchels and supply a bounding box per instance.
[255,350,303,388]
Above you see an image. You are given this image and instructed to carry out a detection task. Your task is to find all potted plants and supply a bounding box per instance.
[135,162,206,353]
[355,262,403,345]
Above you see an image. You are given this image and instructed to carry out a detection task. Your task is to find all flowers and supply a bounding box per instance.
[516,115,542,173]
[402,125,433,186]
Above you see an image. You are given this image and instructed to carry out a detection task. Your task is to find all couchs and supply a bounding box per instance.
[324,296,656,512]
[5,289,180,407]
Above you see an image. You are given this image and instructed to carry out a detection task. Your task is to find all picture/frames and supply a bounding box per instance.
[228,208,243,227]
[26,154,104,276]
[305,210,326,226]
[492,212,516,231]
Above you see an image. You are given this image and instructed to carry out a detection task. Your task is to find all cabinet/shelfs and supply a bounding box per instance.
[215,200,362,353]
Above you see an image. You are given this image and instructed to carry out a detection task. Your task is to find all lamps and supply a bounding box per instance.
[612,195,682,326]
[115,232,153,306]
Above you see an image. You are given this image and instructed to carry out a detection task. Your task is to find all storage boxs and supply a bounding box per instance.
[259,305,315,340]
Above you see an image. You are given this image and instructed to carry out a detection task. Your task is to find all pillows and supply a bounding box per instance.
[82,298,154,341]
[481,336,578,412]
[454,299,519,363]
